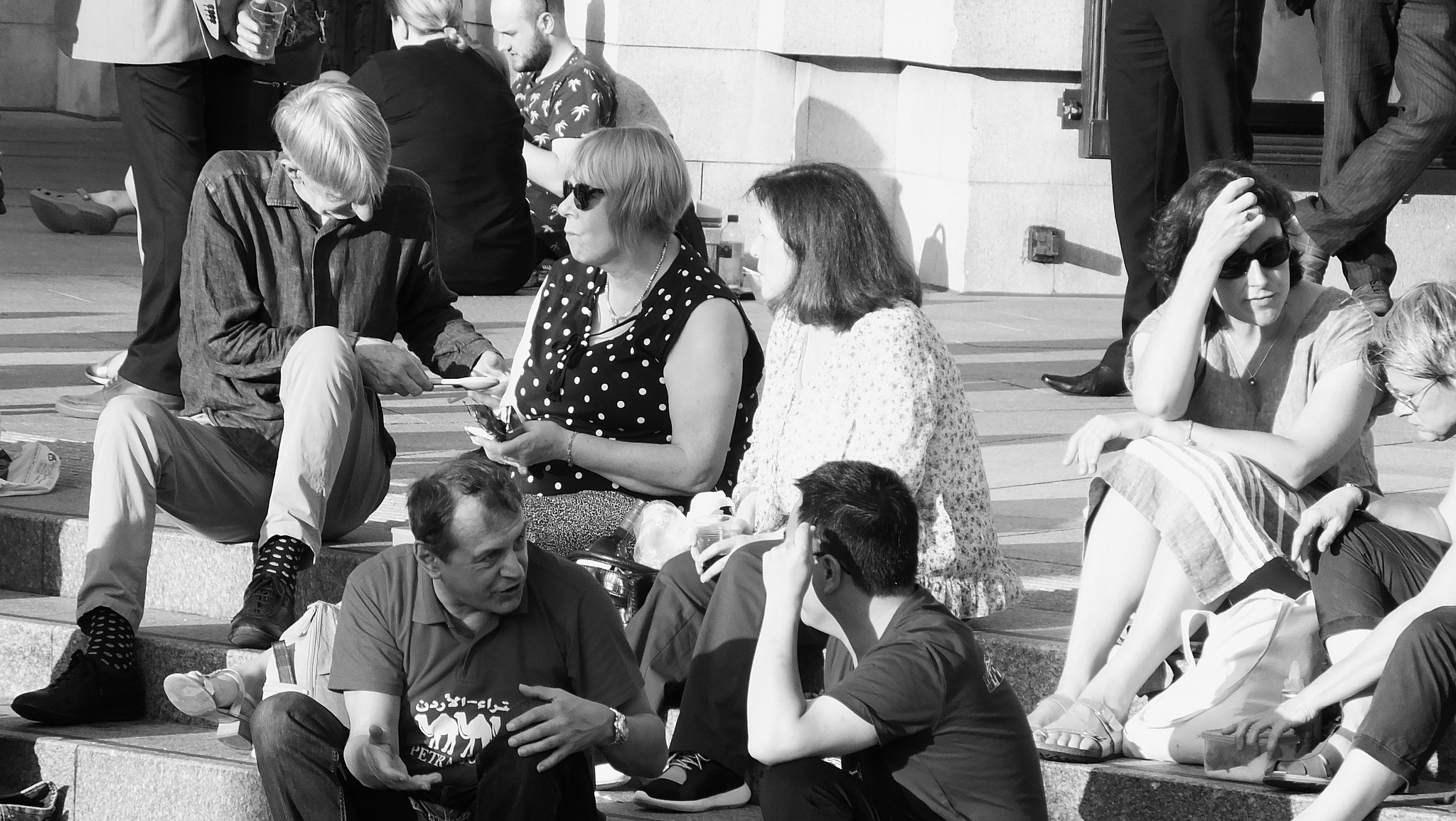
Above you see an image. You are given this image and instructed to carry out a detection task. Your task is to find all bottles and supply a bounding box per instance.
[582,500,648,555]
[719,215,743,287]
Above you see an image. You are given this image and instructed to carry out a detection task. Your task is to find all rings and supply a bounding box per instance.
[504,453,509,462]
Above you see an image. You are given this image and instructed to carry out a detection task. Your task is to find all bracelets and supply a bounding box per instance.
[1185,420,1195,447]
[1340,482,1369,511]
[568,431,578,468]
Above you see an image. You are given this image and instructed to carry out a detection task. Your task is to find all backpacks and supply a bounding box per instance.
[262,598,351,728]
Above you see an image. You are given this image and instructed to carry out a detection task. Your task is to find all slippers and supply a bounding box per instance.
[28,186,119,235]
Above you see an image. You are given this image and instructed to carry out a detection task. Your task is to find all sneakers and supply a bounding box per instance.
[84,349,128,383]
[1284,214,1331,285]
[632,750,752,812]
[593,762,630,790]
[55,374,184,420]
[11,649,149,725]
[1353,280,1394,314]
[228,574,295,646]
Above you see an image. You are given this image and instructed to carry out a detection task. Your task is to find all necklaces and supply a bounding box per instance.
[1223,308,1287,386]
[605,238,668,323]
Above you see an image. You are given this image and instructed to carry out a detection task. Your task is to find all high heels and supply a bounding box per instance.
[163,667,257,755]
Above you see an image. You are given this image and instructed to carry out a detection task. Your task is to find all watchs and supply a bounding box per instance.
[593,706,630,756]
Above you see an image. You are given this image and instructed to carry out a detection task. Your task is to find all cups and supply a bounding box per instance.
[694,515,732,574]
[250,2,287,60]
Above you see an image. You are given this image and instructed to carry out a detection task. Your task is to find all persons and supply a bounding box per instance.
[8,81,507,728]
[8,0,1456,819]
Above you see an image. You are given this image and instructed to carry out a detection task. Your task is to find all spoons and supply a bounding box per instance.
[428,377,500,390]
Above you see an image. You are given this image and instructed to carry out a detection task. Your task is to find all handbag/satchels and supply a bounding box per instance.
[1121,588,1325,766]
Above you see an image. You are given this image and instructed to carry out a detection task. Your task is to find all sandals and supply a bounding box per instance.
[1262,724,1353,787]
[1021,694,1125,761]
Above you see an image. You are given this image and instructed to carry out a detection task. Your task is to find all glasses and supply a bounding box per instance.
[1385,374,1440,412]
[1218,237,1291,280]
[563,179,605,211]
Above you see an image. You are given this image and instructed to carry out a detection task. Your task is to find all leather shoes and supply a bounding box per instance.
[1041,363,1128,397]
